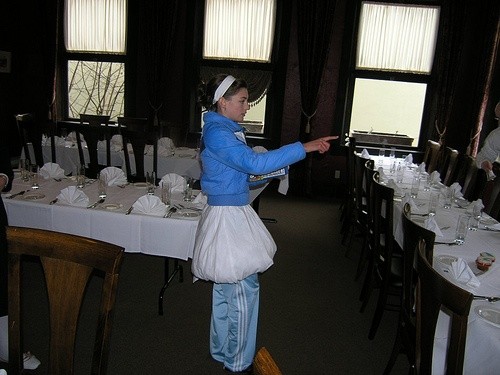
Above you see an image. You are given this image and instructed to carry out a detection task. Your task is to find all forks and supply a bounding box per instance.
[53,178,66,182]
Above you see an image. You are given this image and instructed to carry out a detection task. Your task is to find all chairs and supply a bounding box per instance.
[0,113,157,375]
[344,133,500,375]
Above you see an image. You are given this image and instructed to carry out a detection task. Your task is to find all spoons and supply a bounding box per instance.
[92,199,104,209]
[10,190,25,200]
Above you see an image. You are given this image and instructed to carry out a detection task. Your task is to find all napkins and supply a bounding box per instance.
[131,195,167,216]
[40,162,66,180]
[449,182,464,198]
[386,179,402,195]
[378,167,388,180]
[158,173,190,193]
[430,170,442,185]
[56,185,89,208]
[417,162,425,172]
[447,257,479,290]
[466,199,485,214]
[405,193,419,213]
[424,215,443,238]
[100,167,128,187]
[405,153,413,164]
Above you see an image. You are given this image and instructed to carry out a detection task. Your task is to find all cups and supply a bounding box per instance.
[389,158,396,173]
[71,131,81,143]
[444,188,453,209]
[378,150,385,166]
[423,172,432,192]
[19,158,193,206]
[411,177,421,199]
[428,194,436,217]
[397,166,404,183]
[457,214,471,241]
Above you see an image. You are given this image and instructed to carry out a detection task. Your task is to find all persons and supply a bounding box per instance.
[191,73,340,375]
[475,125,500,182]
[0,72,42,370]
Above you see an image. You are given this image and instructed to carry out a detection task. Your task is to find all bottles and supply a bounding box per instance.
[475,251,495,271]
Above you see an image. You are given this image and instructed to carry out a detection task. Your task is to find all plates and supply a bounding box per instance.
[393,192,498,267]
[179,153,193,157]
[134,183,148,189]
[102,202,121,210]
[178,208,202,218]
[22,192,46,201]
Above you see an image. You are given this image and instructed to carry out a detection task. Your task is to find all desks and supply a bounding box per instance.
[1,174,208,315]
[359,154,500,375]
[20,137,289,224]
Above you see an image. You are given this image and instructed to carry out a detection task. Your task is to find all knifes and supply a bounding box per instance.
[434,242,458,246]
